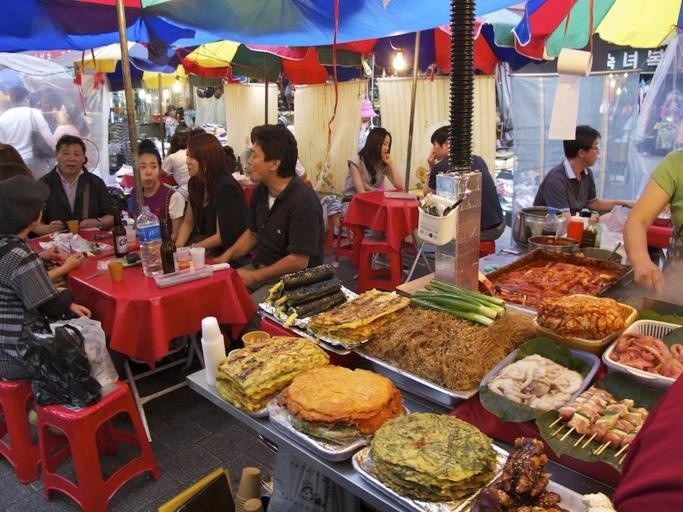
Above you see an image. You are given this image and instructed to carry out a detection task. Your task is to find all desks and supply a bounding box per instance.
[186,367,615,512]
[28,228,258,443]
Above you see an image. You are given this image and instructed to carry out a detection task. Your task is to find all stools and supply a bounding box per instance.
[357,239,403,291]
[33,381,161,512]
[479,239,497,255]
[0,379,66,485]
[333,217,365,269]
[324,213,340,256]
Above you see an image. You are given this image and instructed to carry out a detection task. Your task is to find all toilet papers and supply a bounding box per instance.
[548,48,593,140]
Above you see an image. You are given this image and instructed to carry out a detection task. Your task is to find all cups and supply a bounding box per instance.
[176,246,206,271]
[112,205,130,257]
[233,466,263,510]
[201,316,227,389]
[67,220,79,235]
[108,261,124,281]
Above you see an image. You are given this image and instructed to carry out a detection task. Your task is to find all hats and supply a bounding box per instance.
[0,175,51,231]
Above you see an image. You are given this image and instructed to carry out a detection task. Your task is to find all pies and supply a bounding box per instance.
[216,289,504,501]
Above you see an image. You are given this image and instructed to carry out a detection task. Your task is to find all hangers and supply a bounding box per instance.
[661,88,682,130]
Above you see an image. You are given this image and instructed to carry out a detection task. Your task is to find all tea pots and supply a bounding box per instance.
[513,204,569,247]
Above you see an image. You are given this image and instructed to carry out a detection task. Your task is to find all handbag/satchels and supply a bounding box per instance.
[320,193,341,232]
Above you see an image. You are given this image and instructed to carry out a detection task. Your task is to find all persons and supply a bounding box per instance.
[1,124,325,428]
[533,125,638,219]
[342,125,405,216]
[624,149,683,303]
[422,126,507,240]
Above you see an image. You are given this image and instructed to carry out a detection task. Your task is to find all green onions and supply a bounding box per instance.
[411,278,508,328]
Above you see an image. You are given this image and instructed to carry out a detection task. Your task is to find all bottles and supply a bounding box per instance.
[581,217,602,249]
[136,205,164,278]
[541,208,559,237]
[159,219,175,274]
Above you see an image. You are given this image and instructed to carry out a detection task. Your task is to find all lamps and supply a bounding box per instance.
[138,74,188,105]
[386,37,407,70]
[607,72,629,96]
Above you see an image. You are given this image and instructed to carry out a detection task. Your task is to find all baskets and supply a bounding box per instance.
[533,293,638,356]
[602,319,683,389]
